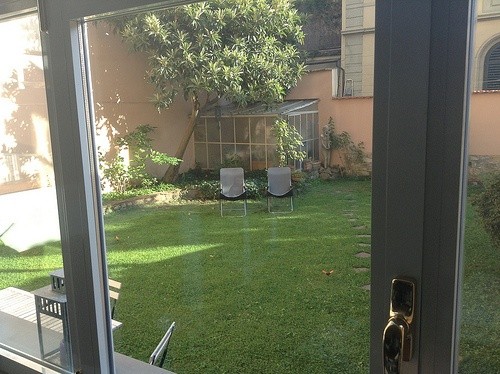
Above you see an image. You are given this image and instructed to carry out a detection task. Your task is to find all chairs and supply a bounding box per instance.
[267,168,293,214]
[149,321,175,368]
[219,167,247,217]
[109,279,121,319]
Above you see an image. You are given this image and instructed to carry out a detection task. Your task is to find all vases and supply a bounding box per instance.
[303,160,313,171]
[312,159,321,169]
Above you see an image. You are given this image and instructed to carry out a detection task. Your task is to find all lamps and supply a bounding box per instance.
[32,269,72,372]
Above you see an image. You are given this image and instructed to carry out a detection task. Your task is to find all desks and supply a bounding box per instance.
[0,287,123,333]
